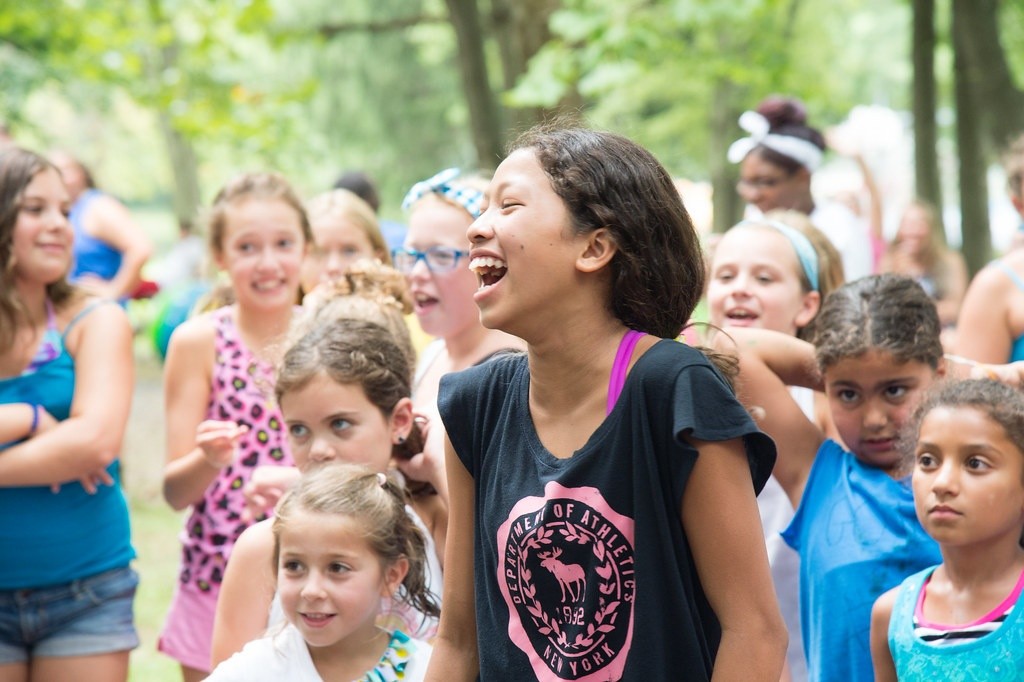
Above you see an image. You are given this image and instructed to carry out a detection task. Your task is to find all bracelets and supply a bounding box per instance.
[27,402,38,434]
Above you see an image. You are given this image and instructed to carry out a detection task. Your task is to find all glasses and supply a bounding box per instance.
[389,246,470,275]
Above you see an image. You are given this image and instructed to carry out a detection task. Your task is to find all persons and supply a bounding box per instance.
[424,126,789,681]
[0,125,150,682]
[869,378,1024,681]
[706,101,1023,682]
[158,168,528,681]
